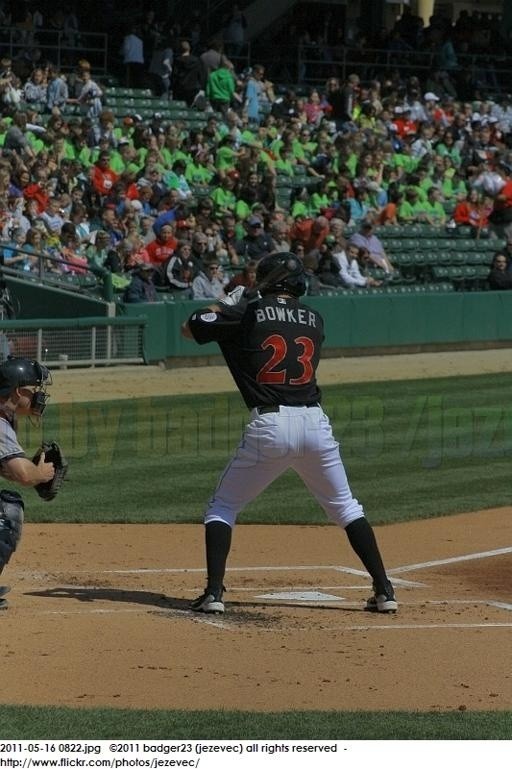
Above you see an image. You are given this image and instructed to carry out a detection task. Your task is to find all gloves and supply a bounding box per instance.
[217,284,246,310]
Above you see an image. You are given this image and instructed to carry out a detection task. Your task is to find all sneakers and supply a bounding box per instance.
[188,589,226,613]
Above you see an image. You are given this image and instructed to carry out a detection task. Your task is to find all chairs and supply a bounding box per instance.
[27,87,512,301]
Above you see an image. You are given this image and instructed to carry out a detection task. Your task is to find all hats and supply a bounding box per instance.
[387,91,500,145]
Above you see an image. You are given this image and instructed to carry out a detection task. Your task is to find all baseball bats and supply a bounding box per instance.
[243,259,298,299]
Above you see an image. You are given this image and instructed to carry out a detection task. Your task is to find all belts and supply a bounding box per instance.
[254,399,321,413]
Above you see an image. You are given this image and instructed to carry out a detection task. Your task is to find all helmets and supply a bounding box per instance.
[254,250,309,298]
[0,355,54,416]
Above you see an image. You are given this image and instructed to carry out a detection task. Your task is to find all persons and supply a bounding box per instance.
[0,354,56,608]
[0,0,512,302]
[179,251,401,614]
